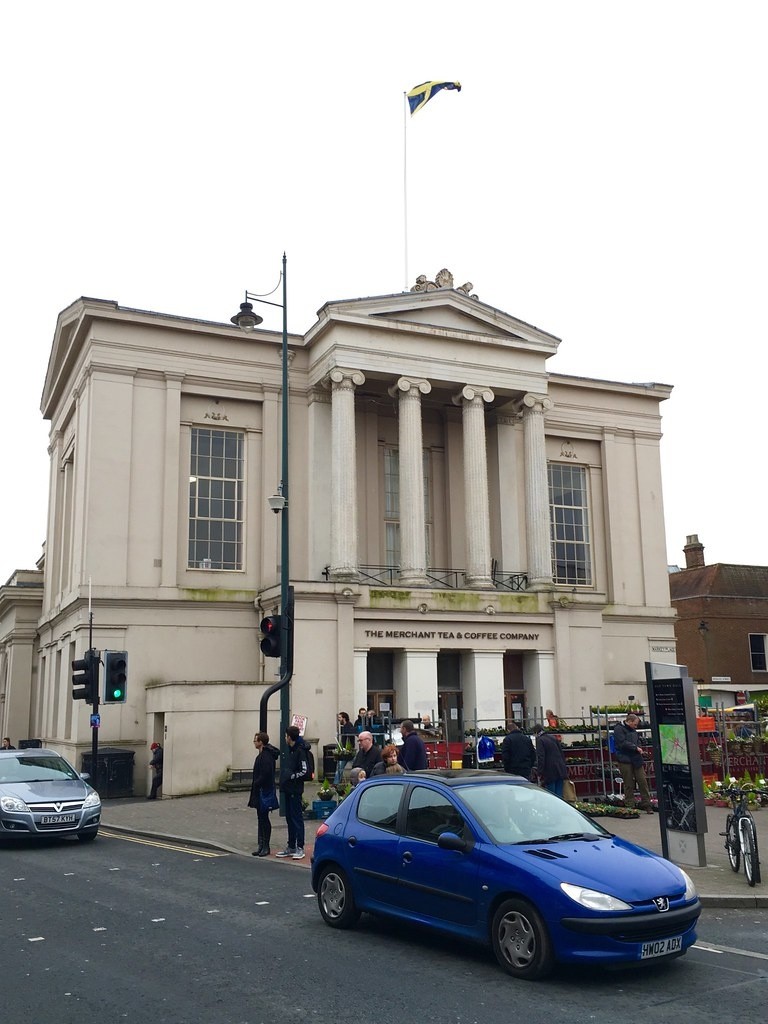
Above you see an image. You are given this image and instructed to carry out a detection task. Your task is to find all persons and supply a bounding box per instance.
[147,742,163,799]
[247,732,280,857]
[502,723,537,779]
[0,737,15,750]
[613,714,654,815]
[532,724,569,800]
[275,726,310,860]
[330,705,440,794]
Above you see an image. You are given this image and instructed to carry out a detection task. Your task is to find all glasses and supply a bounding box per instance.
[357,738,369,742]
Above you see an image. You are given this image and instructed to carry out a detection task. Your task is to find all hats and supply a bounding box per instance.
[151,742,158,750]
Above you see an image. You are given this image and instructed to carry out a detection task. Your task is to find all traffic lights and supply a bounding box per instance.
[104,651,128,705]
[260,616,288,658]
[71,651,101,704]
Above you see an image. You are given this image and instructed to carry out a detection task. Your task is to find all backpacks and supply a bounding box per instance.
[302,750,315,782]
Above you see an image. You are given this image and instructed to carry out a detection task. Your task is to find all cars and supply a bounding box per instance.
[0,748,101,844]
[310,767,702,977]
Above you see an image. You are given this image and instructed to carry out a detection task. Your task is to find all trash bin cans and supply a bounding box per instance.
[322,744,339,782]
[78,746,136,799]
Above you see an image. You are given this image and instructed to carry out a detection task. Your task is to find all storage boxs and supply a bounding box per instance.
[313,801,337,820]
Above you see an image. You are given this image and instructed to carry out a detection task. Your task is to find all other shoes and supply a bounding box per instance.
[252,849,261,855]
[259,848,270,856]
[147,795,157,800]
[647,809,654,814]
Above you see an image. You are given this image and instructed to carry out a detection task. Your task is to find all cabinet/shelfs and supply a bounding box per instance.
[336,714,391,783]
[382,709,451,769]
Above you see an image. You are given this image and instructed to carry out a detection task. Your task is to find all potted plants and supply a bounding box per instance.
[697,692,768,813]
[334,736,357,761]
[336,782,350,796]
[317,776,336,801]
[300,796,309,812]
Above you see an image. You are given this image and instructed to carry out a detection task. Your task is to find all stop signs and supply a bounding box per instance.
[737,693,746,704]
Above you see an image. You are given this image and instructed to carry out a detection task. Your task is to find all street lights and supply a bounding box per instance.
[231,251,296,818]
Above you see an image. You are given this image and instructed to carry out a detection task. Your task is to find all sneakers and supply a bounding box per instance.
[293,847,305,859]
[275,847,297,857]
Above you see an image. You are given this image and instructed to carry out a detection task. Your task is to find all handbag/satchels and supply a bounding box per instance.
[563,770,577,801]
[629,752,644,768]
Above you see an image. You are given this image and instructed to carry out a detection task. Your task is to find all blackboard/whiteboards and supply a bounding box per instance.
[291,714,307,738]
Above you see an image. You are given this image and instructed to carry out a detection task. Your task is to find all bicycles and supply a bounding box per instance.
[714,787,767,887]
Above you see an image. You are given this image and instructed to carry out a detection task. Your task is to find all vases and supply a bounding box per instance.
[465,700,660,818]
